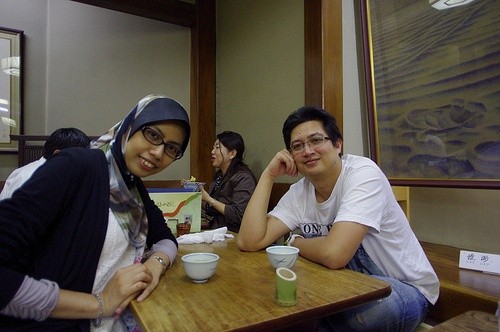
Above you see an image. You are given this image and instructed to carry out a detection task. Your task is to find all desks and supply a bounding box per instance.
[130,230,391,332]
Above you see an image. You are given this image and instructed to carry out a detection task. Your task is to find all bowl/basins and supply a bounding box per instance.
[266,246,299,270]
[182,182,206,192]
[181,253,219,283]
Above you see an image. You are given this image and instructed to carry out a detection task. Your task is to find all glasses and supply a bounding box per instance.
[139,126,184,162]
[289,136,333,153]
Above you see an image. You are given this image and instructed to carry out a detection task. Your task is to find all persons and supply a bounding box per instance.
[199,130,257,234]
[237,105,440,332]
[0,93,190,332]
[0,128,89,201]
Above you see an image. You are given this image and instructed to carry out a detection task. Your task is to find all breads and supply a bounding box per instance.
[190,176,196,182]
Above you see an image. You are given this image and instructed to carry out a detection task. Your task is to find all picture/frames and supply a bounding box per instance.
[0,25,24,152]
[360,0,500,187]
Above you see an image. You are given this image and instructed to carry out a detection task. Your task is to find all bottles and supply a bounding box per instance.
[176,224,190,237]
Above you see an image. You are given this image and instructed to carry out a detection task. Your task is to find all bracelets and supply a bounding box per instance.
[92,294,103,327]
[210,201,214,206]
[156,256,166,273]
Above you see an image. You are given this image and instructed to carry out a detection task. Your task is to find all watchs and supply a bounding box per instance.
[287,235,304,245]
